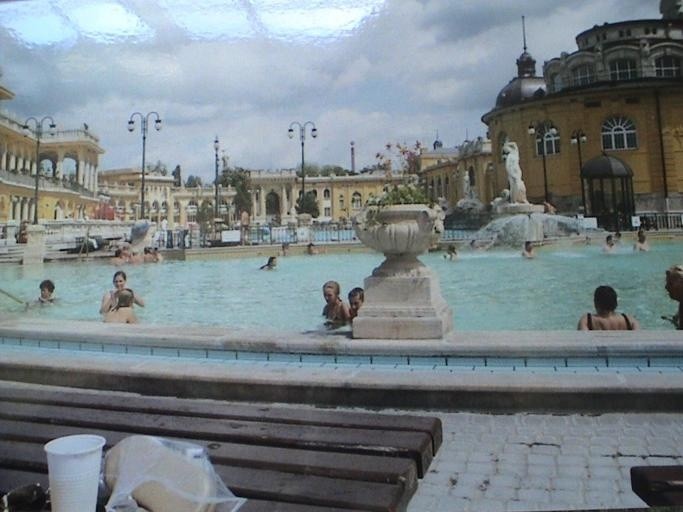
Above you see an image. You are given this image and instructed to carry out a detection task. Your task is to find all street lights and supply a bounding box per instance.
[569,126,590,217]
[527,116,559,207]
[128,110,164,223]
[22,115,59,228]
[213,134,220,224]
[287,119,319,215]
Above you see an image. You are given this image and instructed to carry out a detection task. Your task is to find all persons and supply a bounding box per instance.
[501,140,529,203]
[541,198,555,214]
[98,271,144,314]
[104,288,136,324]
[109,245,162,264]
[238,205,252,246]
[614,232,622,242]
[302,242,327,254]
[461,170,472,199]
[491,188,510,210]
[37,279,55,305]
[603,234,615,254]
[576,285,640,330]
[347,287,363,327]
[663,264,682,330]
[521,240,536,259]
[261,256,278,269]
[277,241,292,257]
[633,230,649,251]
[322,281,351,327]
[446,243,459,262]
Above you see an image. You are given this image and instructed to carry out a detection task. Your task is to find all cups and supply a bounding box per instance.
[44,434,107,512]
